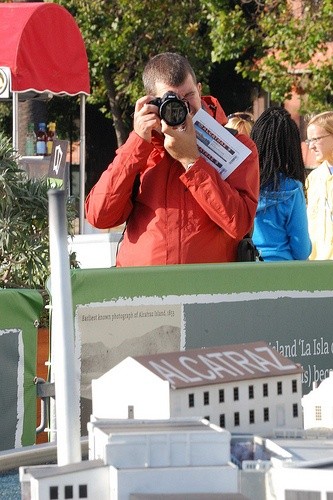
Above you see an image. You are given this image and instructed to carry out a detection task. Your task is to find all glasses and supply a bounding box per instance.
[228,113,255,126]
[304,133,331,145]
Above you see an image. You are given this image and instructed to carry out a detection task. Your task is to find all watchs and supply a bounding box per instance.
[186,161,196,169]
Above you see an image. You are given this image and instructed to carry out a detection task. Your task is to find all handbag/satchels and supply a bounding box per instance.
[225,127,264,262]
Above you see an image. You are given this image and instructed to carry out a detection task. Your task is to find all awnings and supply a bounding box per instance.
[0,3,91,234]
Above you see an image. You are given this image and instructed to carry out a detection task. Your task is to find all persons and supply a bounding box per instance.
[84,50,260,268]
[224,111,256,137]
[305,110,333,260]
[250,107,312,261]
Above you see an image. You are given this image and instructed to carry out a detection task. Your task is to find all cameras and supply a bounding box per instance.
[148,91,190,126]
